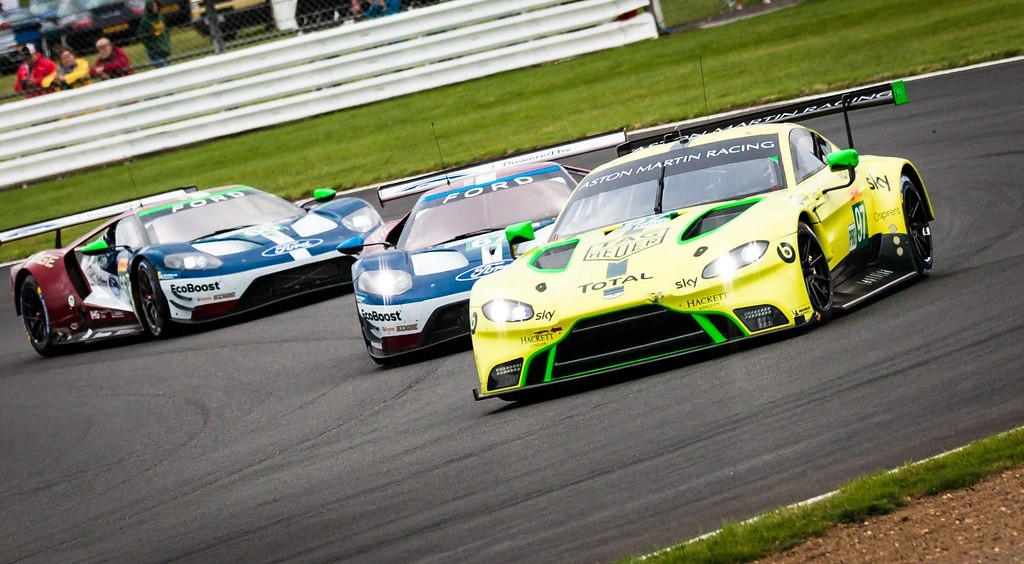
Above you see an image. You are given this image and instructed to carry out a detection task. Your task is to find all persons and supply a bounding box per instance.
[135,0,172,69]
[351,0,402,21]
[41,48,104,122]
[88,38,134,80]
[13,42,65,151]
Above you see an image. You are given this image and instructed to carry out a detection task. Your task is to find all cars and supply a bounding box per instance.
[190,0,351,41]
[0,0,193,77]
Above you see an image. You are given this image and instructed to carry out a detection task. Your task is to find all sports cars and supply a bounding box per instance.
[468,81,936,405]
[0,186,387,358]
[335,127,632,368]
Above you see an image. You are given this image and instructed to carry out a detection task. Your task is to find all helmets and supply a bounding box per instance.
[103,225,116,250]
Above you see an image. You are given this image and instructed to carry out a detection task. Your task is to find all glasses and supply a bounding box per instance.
[96,43,112,51]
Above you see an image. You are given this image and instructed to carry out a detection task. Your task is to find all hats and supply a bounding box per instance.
[21,43,36,55]
